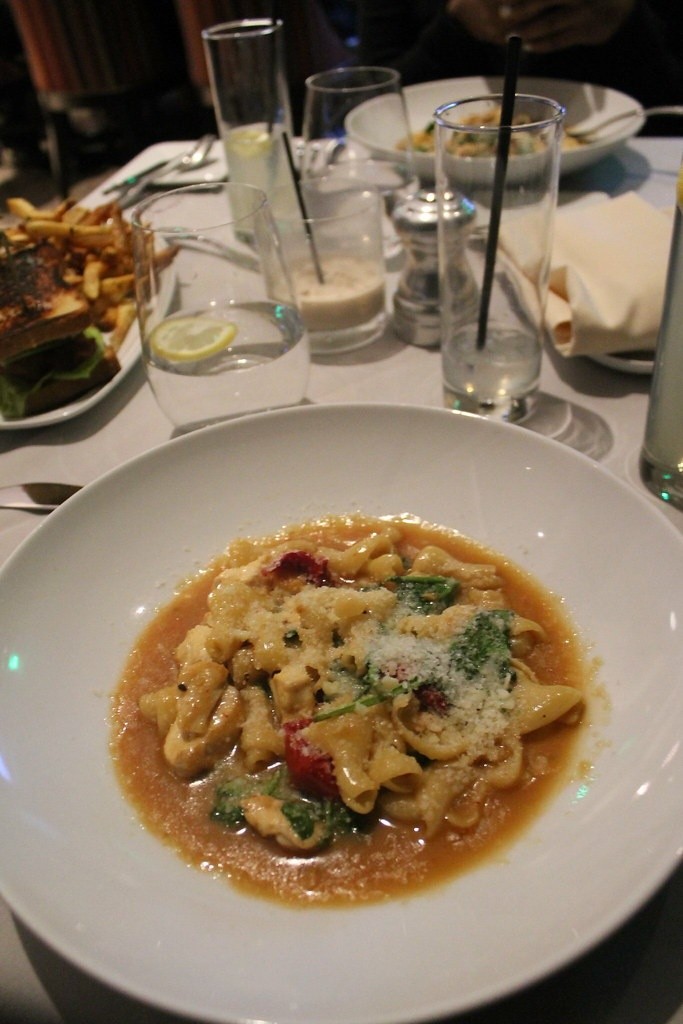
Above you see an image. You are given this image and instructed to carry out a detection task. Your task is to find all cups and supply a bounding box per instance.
[202,18,296,244]
[300,67,420,271]
[639,158,683,512]
[130,183,310,432]
[258,180,387,353]
[433,93,566,425]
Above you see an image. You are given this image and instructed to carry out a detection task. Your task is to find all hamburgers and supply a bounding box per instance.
[0,282,121,420]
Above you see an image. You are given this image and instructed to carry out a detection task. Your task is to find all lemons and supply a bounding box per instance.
[229,131,270,157]
[150,316,235,363]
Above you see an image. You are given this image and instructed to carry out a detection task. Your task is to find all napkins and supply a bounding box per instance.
[493,193,674,358]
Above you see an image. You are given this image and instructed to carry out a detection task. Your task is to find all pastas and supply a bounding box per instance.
[407,107,588,158]
[140,518,582,820]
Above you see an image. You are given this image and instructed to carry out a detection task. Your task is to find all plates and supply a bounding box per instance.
[344,76,645,187]
[589,352,655,373]
[110,139,229,185]
[0,403,683,1024]
[0,218,176,428]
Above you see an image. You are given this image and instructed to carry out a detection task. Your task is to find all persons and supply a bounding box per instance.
[357,0,683,139]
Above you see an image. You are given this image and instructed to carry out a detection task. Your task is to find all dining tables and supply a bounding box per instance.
[0,139,683,1024]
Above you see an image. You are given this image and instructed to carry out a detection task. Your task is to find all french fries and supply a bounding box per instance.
[0,195,181,351]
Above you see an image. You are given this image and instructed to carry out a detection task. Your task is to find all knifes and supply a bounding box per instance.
[1,482,83,508]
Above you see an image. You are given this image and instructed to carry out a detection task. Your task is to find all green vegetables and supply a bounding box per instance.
[210,571,518,846]
[410,120,534,158]
